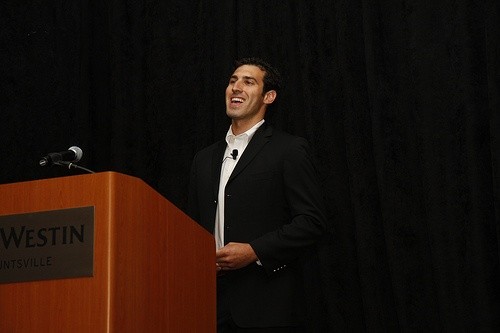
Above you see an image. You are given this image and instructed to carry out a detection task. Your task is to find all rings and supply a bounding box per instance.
[216,263,220,266]
[220,267,224,272]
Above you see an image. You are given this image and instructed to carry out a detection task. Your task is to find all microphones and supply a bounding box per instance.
[232,149,238,159]
[39,146,82,166]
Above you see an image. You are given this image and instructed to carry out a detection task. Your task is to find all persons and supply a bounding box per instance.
[184,56,324,332]
